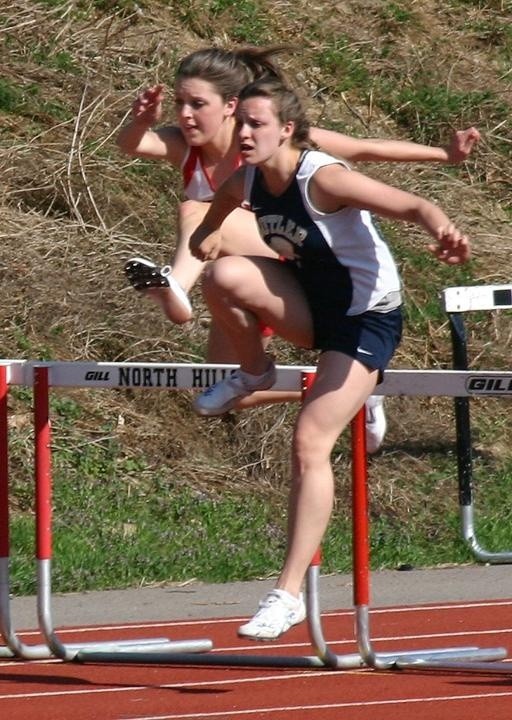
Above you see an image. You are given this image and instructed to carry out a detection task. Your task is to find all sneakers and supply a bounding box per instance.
[365,395,387,454]
[122,257,193,323]
[190,357,277,417]
[237,588,307,642]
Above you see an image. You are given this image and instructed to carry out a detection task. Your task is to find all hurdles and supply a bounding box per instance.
[440,281,512,566]
[0,358,512,676]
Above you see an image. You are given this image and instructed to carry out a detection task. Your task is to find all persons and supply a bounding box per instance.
[190,71,472,643]
[115,44,483,455]
